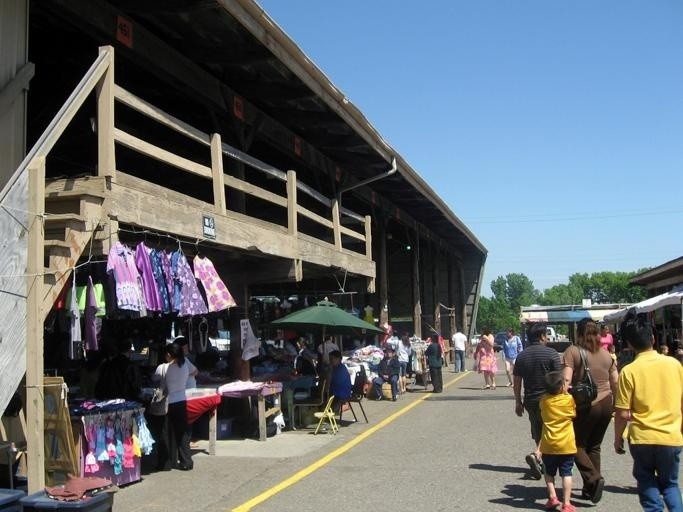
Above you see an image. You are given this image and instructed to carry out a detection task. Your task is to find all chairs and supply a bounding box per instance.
[337,371,368,422]
[290,366,333,430]
[313,396,339,434]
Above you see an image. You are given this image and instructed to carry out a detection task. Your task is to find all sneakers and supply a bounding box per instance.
[591,477,605,503]
[525,453,542,480]
[562,504,577,512]
[376,396,382,401]
[543,497,560,510]
[393,396,396,401]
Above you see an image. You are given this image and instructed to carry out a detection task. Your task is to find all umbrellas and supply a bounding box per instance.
[271,297,390,343]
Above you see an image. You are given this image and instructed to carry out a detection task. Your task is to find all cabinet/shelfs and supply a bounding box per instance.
[222,382,282,441]
[187,388,222,455]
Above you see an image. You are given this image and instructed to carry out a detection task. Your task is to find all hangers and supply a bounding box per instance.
[193,243,212,263]
[88,263,93,287]
[364,302,374,312]
[113,229,133,250]
[108,408,126,423]
[132,407,141,419]
[136,232,174,255]
[173,239,185,259]
[72,267,77,286]
[87,412,94,429]
[98,411,104,426]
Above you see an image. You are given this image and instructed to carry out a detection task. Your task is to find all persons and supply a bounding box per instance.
[513,322,563,480]
[423,333,443,394]
[79,351,101,393]
[560,318,618,504]
[598,322,613,352]
[393,330,398,345]
[614,322,683,511]
[659,344,668,357]
[608,343,618,367]
[617,340,635,373]
[318,350,351,418]
[150,342,198,471]
[395,332,412,396]
[110,339,141,400]
[172,338,198,448]
[294,335,319,398]
[674,348,683,362]
[426,331,447,360]
[384,333,394,351]
[473,325,497,390]
[539,372,577,512]
[502,326,523,387]
[451,327,468,373]
[318,335,339,397]
[372,346,400,402]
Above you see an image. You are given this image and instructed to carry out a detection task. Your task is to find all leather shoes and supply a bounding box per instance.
[175,464,188,470]
[484,385,490,389]
[492,384,496,390]
[505,382,512,387]
[188,464,193,470]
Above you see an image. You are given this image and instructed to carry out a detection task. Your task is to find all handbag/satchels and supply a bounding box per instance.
[149,363,169,415]
[569,347,597,405]
